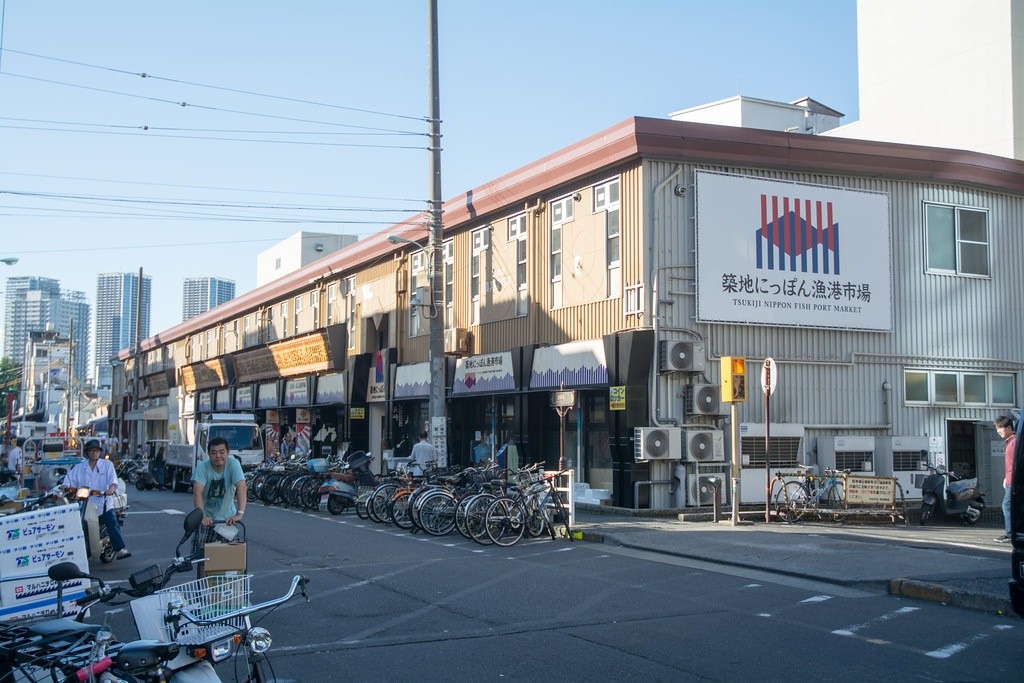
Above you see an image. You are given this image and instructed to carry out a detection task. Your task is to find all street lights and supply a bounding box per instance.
[384,237,446,473]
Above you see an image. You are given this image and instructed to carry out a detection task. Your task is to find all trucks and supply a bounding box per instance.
[163,414,265,492]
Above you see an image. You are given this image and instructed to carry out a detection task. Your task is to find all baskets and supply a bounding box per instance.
[514,471,539,496]
[474,467,499,481]
[425,467,447,485]
[154,575,254,645]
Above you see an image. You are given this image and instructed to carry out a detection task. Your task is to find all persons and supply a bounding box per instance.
[137,444,144,456]
[100,439,109,459]
[1,433,17,470]
[994,416,1017,543]
[408,430,436,487]
[155,446,167,491]
[281,432,295,459]
[190,436,248,604]
[8,437,26,471]
[50,439,128,556]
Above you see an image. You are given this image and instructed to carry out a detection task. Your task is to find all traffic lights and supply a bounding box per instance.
[719,353,747,402]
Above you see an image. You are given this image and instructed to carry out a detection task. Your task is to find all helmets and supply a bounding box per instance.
[84,439,103,454]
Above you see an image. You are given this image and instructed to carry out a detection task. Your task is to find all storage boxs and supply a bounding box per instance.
[112,494,127,508]
[200,565,244,606]
[203,542,246,571]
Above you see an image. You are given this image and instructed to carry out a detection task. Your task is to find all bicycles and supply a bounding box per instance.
[232,449,575,548]
[773,463,852,522]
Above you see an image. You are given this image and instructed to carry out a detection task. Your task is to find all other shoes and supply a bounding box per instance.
[993,535,1011,542]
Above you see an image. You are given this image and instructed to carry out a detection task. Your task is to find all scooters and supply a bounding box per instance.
[0,439,313,683]
[921,447,986,525]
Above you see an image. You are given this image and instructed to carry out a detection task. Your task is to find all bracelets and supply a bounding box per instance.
[237,510,244,514]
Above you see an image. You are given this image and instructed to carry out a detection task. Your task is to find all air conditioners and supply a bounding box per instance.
[634,427,681,460]
[444,326,468,354]
[681,430,725,462]
[686,384,731,415]
[686,473,727,505]
[659,339,705,372]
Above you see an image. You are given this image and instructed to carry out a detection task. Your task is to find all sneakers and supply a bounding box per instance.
[116,548,132,560]
